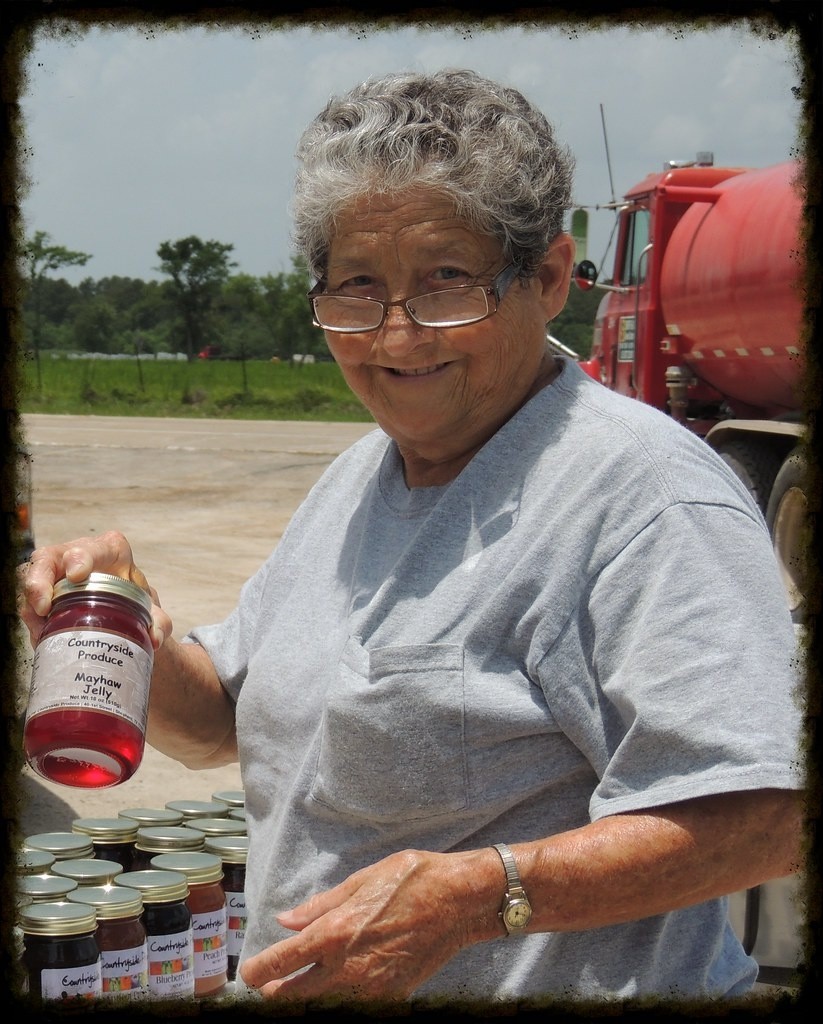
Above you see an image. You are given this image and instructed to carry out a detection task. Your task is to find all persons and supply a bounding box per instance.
[17,72,807,1000]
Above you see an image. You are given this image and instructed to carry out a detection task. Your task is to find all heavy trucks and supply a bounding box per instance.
[571,101,808,605]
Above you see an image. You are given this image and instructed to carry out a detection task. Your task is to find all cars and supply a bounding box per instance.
[199,345,222,358]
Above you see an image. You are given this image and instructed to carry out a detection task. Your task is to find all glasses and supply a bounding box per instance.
[305,247,524,334]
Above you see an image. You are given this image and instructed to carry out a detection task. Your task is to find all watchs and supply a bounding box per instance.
[492,842,532,939]
